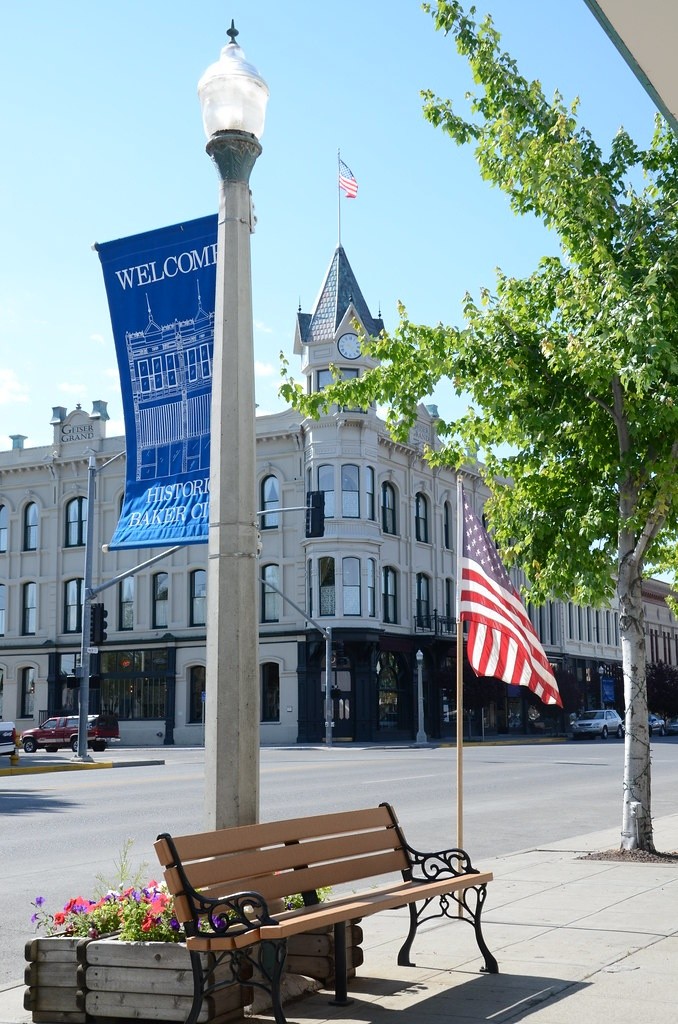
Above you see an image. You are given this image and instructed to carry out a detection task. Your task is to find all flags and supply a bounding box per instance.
[339,158,358,198]
[461,486,564,709]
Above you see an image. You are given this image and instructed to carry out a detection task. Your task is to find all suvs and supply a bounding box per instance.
[21,714,121,754]
[572,709,624,740]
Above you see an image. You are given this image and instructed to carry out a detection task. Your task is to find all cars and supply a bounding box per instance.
[648,713,665,738]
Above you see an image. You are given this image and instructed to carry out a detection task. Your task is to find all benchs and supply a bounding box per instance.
[153,802,499,1024]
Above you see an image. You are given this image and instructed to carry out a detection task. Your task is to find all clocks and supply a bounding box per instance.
[337,333,362,360]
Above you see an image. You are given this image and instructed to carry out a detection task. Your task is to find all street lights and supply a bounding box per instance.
[199,16,262,833]
[415,650,427,742]
